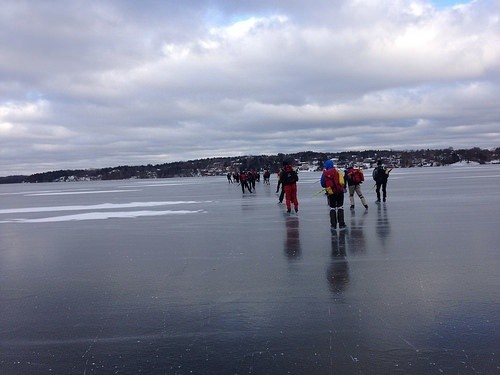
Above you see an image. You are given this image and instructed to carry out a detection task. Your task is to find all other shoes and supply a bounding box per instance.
[350,205,355,210]
[364,204,369,210]
[330,224,337,230]
[383,198,387,203]
[295,209,298,214]
[286,208,291,213]
[374,199,381,204]
[339,223,347,231]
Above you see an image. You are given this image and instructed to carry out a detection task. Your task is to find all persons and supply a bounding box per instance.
[280,203,391,298]
[226,168,286,193]
[320,160,347,229]
[278,175,294,204]
[282,164,299,213]
[372,160,389,203]
[343,162,369,210]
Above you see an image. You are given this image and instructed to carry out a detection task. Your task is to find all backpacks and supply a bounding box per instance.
[281,171,298,185]
[376,167,387,184]
[322,168,345,196]
[348,168,364,184]
